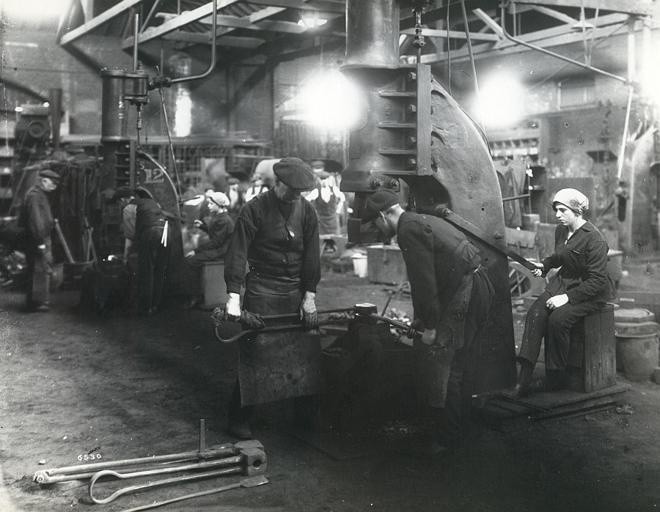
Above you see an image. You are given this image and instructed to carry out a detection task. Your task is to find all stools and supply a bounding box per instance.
[544,306,616,395]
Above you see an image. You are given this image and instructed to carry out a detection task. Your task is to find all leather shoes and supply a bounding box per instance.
[31,305,49,312]
[501,383,529,402]
[181,295,202,312]
[229,420,253,440]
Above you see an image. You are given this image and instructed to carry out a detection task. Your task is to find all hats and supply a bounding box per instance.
[317,172,329,179]
[552,188,589,215]
[251,176,262,183]
[361,191,399,224]
[310,161,324,168]
[227,178,240,185]
[106,189,129,205]
[39,169,61,184]
[209,192,230,207]
[273,157,314,193]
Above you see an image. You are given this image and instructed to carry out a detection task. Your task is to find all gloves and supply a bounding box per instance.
[224,292,241,323]
[300,290,320,335]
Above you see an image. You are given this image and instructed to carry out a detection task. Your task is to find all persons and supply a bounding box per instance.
[224,158,321,440]
[178,156,354,306]
[19,170,61,310]
[113,186,168,316]
[499,187,616,399]
[359,188,496,458]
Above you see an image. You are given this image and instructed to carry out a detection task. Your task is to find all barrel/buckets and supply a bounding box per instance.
[616,324,660,382]
